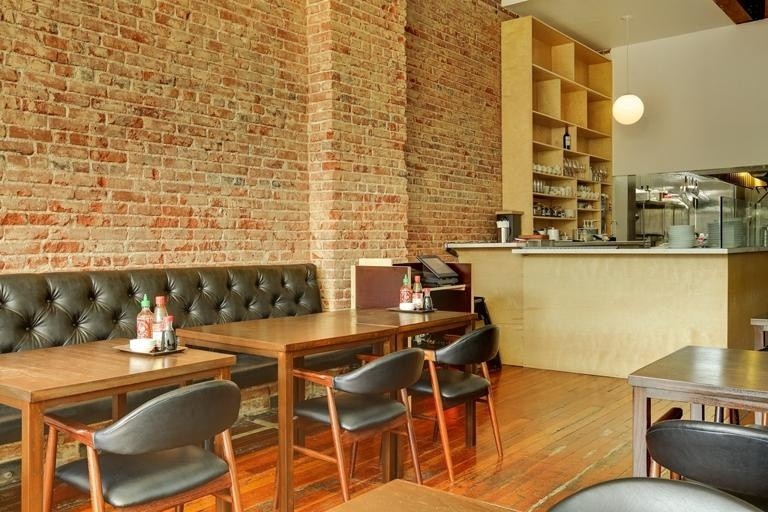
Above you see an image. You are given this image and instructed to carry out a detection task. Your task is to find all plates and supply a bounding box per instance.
[666,225,696,247]
[111,342,187,356]
[635,191,649,201]
[705,223,746,247]
[387,306,437,314]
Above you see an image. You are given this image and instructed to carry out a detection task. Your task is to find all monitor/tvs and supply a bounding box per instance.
[416,254,459,279]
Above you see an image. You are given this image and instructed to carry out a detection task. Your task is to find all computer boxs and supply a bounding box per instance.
[413,272,459,287]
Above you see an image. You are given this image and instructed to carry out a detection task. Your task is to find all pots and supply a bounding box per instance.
[548,229,560,241]
[533,202,545,216]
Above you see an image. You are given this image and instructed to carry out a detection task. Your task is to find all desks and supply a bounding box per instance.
[325,477,528,511]
[1,306,479,511]
[1,336,238,512]
[176,304,478,509]
[627,342,768,478]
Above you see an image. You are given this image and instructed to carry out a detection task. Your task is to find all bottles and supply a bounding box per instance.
[562,124,571,149]
[400,274,433,310]
[760,227,767,248]
[641,185,651,201]
[577,201,593,209]
[137,293,177,352]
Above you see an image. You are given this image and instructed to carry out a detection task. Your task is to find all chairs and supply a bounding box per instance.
[544,478,766,512]
[406,325,505,486]
[43,378,248,512]
[639,407,768,498]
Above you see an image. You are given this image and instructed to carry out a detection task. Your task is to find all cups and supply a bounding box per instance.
[561,211,565,217]
[531,155,608,183]
[532,178,600,200]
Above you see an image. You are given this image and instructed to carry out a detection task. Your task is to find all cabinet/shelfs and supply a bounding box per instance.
[499,14,615,237]
[356,264,471,394]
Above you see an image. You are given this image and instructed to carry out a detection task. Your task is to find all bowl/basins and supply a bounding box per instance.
[129,338,156,354]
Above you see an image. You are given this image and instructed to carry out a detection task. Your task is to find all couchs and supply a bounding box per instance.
[0,262,376,459]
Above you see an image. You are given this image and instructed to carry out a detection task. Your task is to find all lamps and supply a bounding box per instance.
[612,16,645,126]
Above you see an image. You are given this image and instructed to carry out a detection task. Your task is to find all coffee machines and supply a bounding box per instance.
[495,211,523,243]
